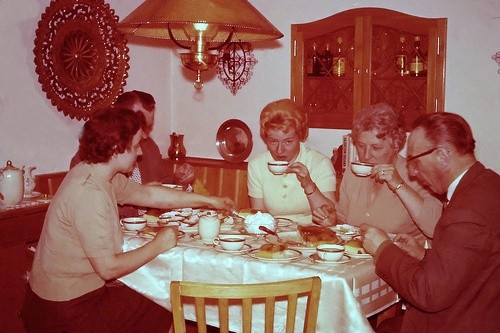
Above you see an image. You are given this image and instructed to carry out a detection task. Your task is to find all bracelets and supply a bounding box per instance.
[392,182,404,193]
[304,183,316,195]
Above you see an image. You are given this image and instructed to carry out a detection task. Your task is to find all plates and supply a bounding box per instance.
[331,226,397,258]
[216,119,253,163]
[248,249,303,261]
[137,231,185,239]
[275,218,293,227]
[310,254,351,264]
[215,243,252,253]
[264,231,343,250]
[23,191,40,198]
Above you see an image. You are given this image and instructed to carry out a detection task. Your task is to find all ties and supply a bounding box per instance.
[128,161,147,215]
[442,192,449,209]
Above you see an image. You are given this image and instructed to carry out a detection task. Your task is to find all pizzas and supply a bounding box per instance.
[343,239,366,254]
[141,225,163,236]
[257,243,288,259]
[296,225,338,247]
[239,208,257,217]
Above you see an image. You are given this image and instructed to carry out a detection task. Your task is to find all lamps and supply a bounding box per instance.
[115,0,285,90]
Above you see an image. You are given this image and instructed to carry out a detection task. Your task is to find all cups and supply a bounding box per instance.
[213,238,245,250]
[317,244,345,261]
[119,217,147,230]
[351,162,374,176]
[268,160,291,174]
[199,216,220,241]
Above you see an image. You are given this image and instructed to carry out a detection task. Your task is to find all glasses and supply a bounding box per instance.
[406,148,437,167]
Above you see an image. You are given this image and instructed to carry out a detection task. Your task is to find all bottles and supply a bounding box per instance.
[19,166,37,196]
[308,36,355,76]
[395,34,429,77]
[168,135,186,159]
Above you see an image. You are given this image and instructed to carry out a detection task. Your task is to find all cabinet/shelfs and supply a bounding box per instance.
[289,6,447,134]
[0,192,52,333]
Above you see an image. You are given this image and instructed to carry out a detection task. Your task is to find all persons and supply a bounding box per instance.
[248,98,336,224]
[23,108,198,333]
[70,90,194,219]
[312,102,443,245]
[360,111,500,333]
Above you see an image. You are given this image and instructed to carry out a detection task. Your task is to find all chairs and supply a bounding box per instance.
[168,277,322,333]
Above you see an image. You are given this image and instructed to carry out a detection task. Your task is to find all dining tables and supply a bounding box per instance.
[117,211,402,333]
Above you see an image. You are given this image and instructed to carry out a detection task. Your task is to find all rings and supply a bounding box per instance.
[382,170,384,175]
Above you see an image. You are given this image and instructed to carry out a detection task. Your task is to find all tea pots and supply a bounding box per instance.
[0,160,25,205]
